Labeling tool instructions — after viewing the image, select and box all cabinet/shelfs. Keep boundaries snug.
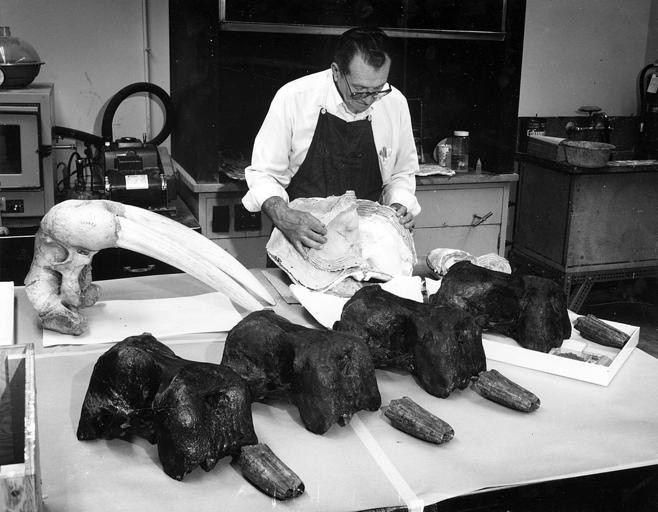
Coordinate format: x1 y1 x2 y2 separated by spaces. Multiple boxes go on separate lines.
171 158 516 278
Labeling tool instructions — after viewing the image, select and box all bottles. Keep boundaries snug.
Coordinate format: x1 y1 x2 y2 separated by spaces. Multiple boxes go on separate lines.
452 131 469 173
476 158 481 173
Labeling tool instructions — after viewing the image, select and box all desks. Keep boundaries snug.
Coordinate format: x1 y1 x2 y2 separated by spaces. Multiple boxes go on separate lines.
30 255 658 512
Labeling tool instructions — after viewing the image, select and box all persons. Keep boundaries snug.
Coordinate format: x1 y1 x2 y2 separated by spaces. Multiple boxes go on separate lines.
242 25 422 269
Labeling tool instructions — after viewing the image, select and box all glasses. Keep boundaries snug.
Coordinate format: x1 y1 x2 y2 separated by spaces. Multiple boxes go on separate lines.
342 69 392 101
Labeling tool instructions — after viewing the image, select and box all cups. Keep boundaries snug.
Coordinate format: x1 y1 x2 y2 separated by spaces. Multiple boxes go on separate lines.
436 144 452 167
